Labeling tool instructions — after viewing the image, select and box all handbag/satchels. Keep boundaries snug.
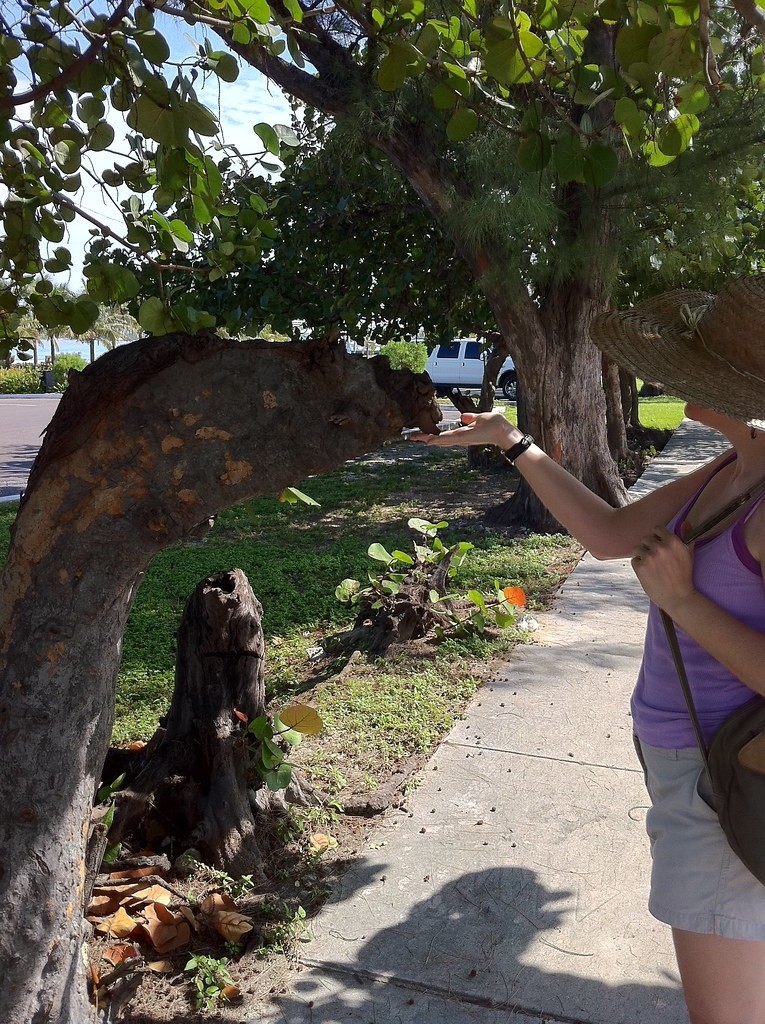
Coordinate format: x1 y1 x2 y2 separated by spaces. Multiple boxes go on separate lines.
706 694 765 887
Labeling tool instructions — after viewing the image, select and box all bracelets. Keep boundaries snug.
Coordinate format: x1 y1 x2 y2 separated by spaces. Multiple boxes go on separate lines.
501 435 534 466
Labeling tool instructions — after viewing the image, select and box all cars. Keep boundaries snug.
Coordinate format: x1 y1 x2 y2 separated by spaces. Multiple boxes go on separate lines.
424 339 517 400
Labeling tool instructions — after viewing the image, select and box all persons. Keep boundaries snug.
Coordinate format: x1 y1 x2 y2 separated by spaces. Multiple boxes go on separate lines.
405 274 764 1023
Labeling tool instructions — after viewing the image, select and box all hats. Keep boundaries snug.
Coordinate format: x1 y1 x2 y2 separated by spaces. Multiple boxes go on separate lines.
589 271 765 420
